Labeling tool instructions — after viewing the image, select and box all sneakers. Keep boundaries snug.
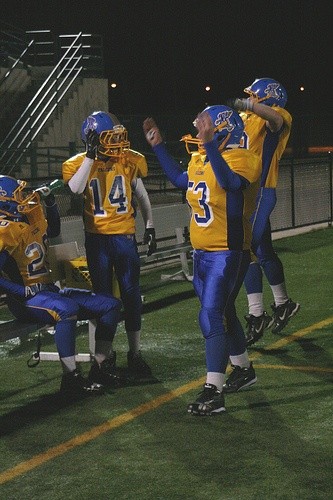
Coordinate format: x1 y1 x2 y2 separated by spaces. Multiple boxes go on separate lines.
126 349 153 376
60 370 105 395
186 383 226 417
270 297 301 334
87 359 128 388
245 311 274 348
220 360 257 392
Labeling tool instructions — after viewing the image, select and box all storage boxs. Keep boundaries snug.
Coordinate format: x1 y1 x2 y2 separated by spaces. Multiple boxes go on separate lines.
64 256 121 298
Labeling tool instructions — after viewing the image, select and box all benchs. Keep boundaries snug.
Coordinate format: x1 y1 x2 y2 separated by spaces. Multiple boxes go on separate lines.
0 243 147 361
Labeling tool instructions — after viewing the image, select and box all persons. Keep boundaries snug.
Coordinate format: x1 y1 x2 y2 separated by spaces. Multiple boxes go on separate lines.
62 111 157 378
142 106 262 415
226 78 301 344
0 175 129 398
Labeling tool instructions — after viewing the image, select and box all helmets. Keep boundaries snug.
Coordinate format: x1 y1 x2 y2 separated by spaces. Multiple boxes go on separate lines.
193 105 245 150
79 109 124 153
0 175 27 220
244 77 288 110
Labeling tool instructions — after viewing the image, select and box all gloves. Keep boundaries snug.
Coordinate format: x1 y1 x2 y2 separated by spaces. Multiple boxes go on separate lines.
206 97 254 113
37 182 56 206
23 282 62 298
141 227 157 258
85 127 100 160
